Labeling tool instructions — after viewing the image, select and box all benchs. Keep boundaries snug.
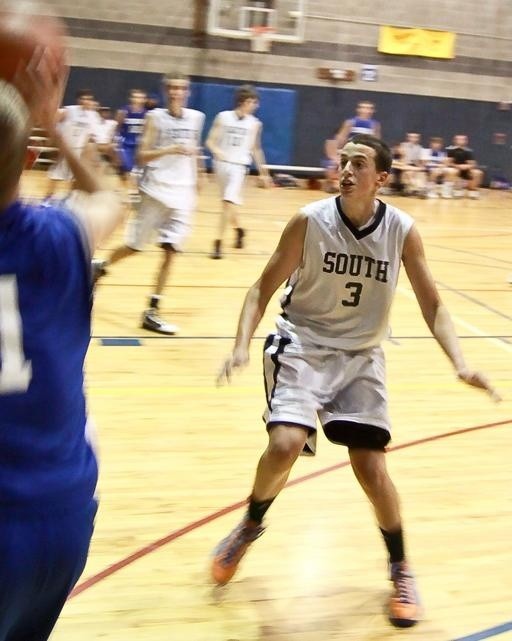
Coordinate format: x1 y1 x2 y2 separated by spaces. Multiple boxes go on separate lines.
26 124 214 174
259 163 329 191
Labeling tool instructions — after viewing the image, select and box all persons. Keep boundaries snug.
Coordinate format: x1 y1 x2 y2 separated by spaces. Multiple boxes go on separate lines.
0 47 127 641
40 71 206 335
328 100 482 199
206 86 273 259
209 133 503 626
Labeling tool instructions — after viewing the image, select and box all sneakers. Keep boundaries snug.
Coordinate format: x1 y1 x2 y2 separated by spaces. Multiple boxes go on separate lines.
385 562 424 629
210 250 222 260
209 517 266 588
141 308 180 335
91 259 106 293
233 230 246 249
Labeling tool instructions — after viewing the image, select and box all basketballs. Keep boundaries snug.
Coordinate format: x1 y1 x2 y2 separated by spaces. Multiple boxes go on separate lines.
0 4 68 107
